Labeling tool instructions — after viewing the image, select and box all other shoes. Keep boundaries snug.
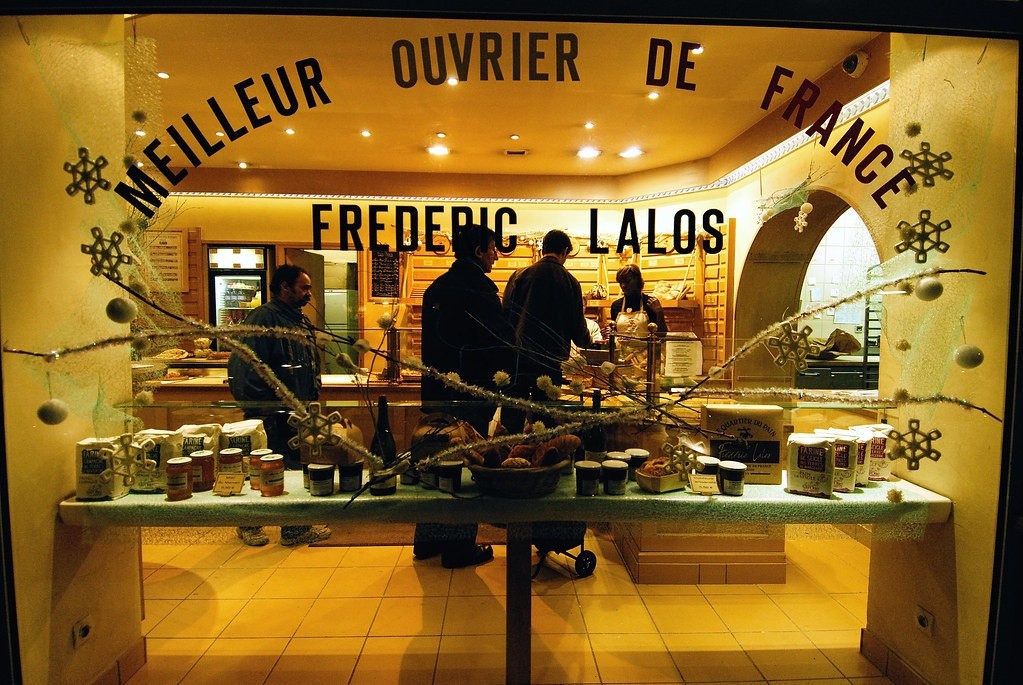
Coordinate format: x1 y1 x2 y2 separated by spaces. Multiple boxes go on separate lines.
234 526 269 546
280 524 331 546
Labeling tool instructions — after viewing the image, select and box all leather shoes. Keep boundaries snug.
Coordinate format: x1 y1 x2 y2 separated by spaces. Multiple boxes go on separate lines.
442 544 493 568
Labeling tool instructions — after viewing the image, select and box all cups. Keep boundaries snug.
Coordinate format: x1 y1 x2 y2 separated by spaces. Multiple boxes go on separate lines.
625 449 649 481
607 451 631 462
602 460 628 495
575 461 602 496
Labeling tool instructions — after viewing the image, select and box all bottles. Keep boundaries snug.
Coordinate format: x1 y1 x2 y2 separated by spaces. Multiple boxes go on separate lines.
719 461 747 496
400 467 418 485
583 390 608 484
259 454 284 497
438 461 463 492
225 283 262 308
249 449 273 490
420 465 438 490
303 464 334 496
219 448 243 474
338 464 363 492
368 396 396 496
166 456 193 499
696 455 721 475
595 340 608 350
190 450 214 492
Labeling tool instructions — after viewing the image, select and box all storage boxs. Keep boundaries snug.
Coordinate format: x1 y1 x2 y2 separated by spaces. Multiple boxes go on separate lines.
635 468 686 493
700 403 783 486
324 261 358 289
659 341 703 378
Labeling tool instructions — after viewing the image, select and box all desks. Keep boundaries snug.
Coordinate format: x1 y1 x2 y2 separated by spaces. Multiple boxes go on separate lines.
58 473 951 685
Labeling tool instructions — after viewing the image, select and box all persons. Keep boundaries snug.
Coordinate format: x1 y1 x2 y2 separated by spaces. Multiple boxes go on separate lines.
501 230 604 434
609 264 668 382
227 264 332 546
412 223 503 569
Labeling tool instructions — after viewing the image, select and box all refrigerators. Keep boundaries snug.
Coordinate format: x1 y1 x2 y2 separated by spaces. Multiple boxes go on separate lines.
324 289 359 374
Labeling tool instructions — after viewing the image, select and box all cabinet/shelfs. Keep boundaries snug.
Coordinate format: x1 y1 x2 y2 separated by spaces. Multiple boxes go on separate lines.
795 355 879 390
